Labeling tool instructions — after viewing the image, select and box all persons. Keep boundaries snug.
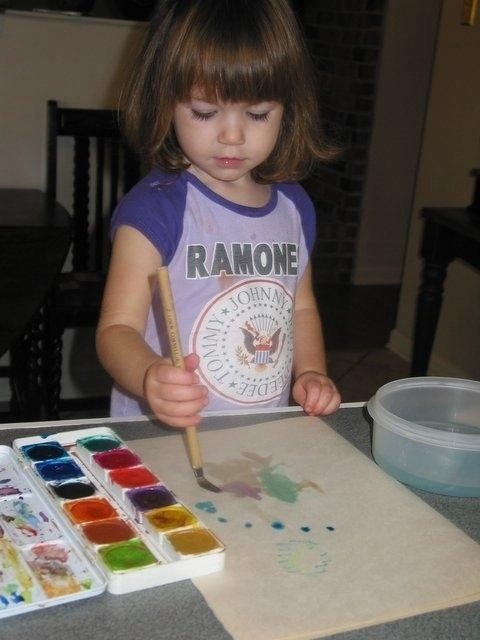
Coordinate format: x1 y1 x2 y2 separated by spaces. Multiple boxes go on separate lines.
94 0 345 429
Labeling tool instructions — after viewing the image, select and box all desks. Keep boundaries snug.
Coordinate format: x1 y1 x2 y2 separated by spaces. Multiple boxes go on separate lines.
0 188 72 420
0 393 480 640
410 169 480 379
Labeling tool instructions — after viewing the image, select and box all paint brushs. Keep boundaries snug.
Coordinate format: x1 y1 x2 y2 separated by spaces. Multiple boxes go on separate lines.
156 265 225 493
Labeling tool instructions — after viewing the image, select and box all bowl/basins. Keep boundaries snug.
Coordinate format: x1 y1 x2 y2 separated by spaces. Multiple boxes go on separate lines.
367 377 480 497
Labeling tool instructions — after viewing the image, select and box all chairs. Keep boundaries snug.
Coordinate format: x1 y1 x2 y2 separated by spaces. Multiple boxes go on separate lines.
29 100 170 420
